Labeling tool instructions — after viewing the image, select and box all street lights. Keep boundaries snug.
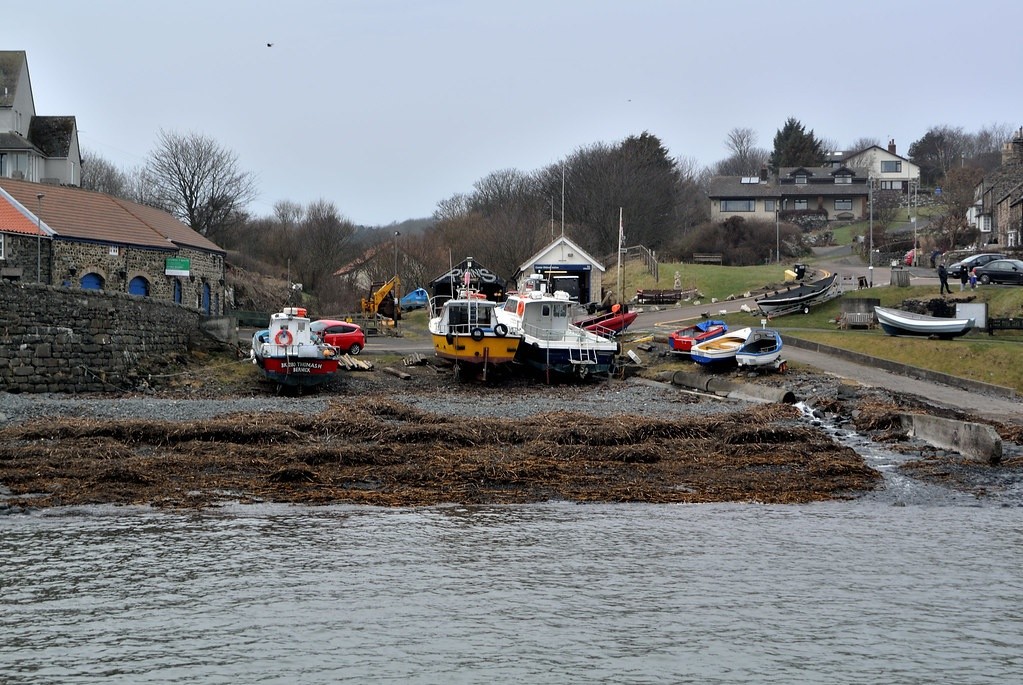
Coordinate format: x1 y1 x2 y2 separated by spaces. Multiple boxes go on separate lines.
909 181 917 266
868 179 875 287
37 193 45 282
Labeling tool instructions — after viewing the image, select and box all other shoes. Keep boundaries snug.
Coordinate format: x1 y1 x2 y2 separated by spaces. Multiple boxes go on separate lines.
941 293 946 295
948 292 954 294
971 289 973 291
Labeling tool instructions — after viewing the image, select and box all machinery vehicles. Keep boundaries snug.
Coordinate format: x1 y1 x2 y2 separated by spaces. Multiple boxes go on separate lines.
359 275 402 334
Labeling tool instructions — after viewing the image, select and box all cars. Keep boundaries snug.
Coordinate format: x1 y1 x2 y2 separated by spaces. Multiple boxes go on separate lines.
972 259 1023 284
309 320 364 355
946 253 1009 279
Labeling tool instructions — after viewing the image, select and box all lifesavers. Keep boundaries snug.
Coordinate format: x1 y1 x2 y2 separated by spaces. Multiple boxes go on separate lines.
275 331 293 348
471 328 484 341
494 323 508 338
446 333 454 345
517 301 524 315
471 294 486 299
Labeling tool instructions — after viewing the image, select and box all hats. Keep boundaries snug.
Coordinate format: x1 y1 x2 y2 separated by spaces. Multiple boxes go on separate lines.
971 268 976 272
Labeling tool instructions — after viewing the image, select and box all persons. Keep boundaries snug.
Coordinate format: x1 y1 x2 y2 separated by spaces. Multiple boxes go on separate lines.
959 263 977 292
988 238 998 244
938 263 953 295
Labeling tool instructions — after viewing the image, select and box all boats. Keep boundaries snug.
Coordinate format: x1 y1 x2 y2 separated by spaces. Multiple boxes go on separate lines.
491 292 620 379
690 327 753 368
735 327 782 367
251 307 340 390
429 271 521 378
758 272 837 319
573 311 637 336
668 319 728 354
873 306 975 338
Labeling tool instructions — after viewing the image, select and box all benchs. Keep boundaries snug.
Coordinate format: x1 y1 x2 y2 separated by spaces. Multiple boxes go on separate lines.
840 312 874 331
706 346 721 350
638 290 682 304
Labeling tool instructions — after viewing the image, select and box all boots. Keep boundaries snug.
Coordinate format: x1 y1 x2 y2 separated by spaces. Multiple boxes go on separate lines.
960 284 966 291
